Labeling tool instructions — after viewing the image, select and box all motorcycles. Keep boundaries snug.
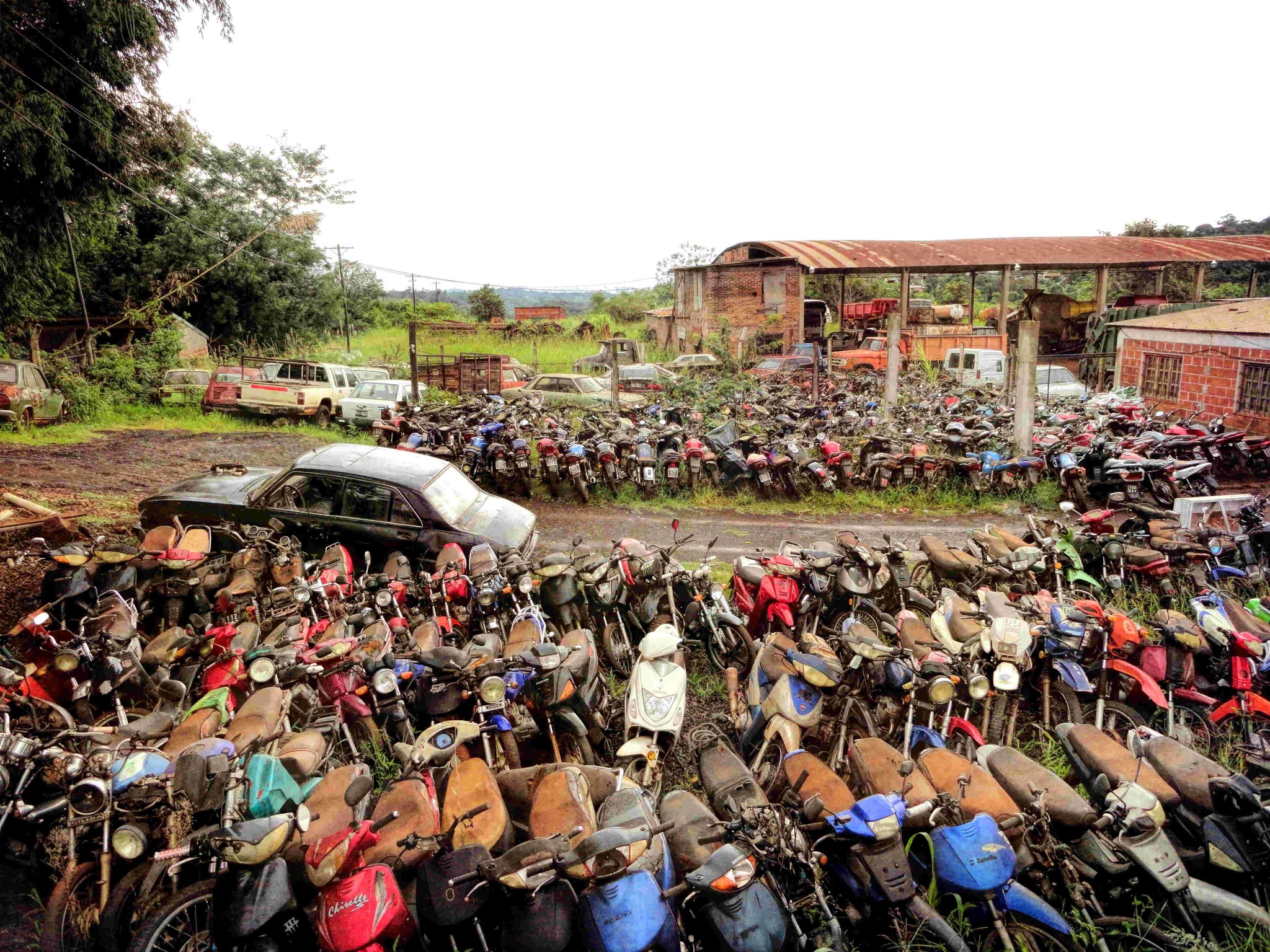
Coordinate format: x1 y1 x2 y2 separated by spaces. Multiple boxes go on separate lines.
0 345 1269 952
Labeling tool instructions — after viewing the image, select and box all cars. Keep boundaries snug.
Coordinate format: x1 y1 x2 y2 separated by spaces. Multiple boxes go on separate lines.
741 353 814 379
673 353 717 362
498 372 650 414
1035 363 1091 400
200 363 268 419
137 442 540 576
0 357 69 432
349 366 389 387
593 362 685 393
782 343 823 373
157 367 214 411
333 377 429 432
259 363 281 380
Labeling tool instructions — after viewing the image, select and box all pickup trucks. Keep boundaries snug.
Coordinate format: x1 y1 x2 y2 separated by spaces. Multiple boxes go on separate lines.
234 360 361 431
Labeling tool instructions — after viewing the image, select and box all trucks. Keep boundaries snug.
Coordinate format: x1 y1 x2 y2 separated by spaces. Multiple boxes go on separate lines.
941 347 1007 391
417 351 543 401
570 336 756 382
825 328 1009 376
804 299 828 343
838 297 899 333
